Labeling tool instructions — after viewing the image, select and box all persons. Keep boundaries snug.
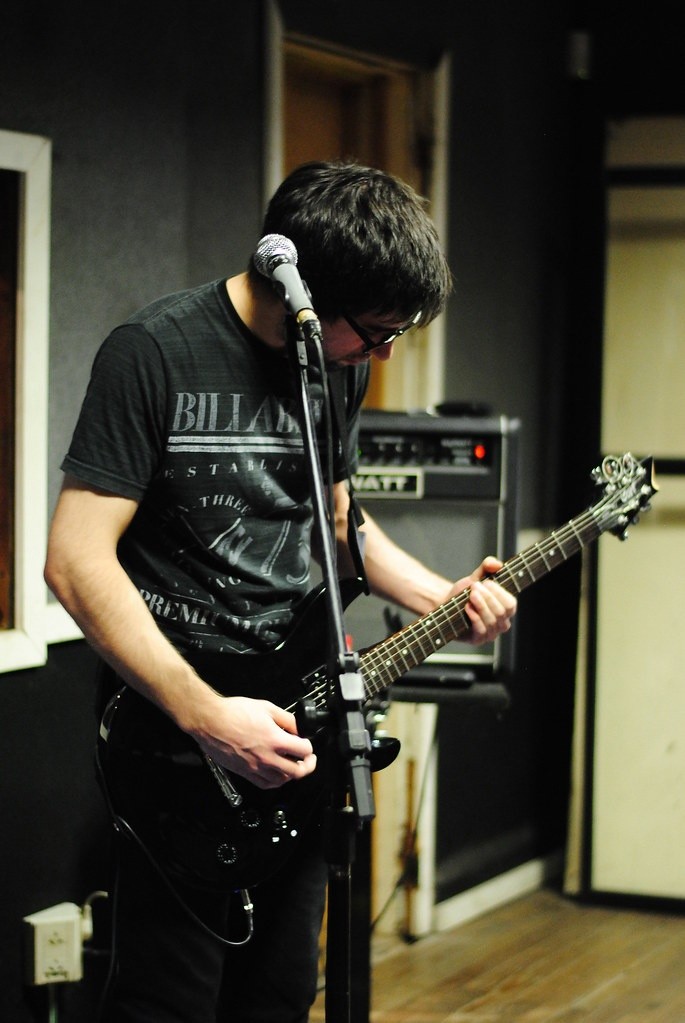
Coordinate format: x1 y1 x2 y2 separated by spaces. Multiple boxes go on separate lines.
42 161 517 1022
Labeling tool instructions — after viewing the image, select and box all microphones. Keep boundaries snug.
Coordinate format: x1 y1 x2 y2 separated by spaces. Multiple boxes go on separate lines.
253 234 321 340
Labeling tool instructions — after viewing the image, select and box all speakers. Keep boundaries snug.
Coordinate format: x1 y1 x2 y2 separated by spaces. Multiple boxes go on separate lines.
340 418 521 686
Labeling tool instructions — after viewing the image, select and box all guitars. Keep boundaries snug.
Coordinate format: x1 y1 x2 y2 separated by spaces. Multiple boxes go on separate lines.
96 448 663 950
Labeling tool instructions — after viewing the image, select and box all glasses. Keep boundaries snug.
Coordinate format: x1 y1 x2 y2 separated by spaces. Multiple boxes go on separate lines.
337 309 423 354
